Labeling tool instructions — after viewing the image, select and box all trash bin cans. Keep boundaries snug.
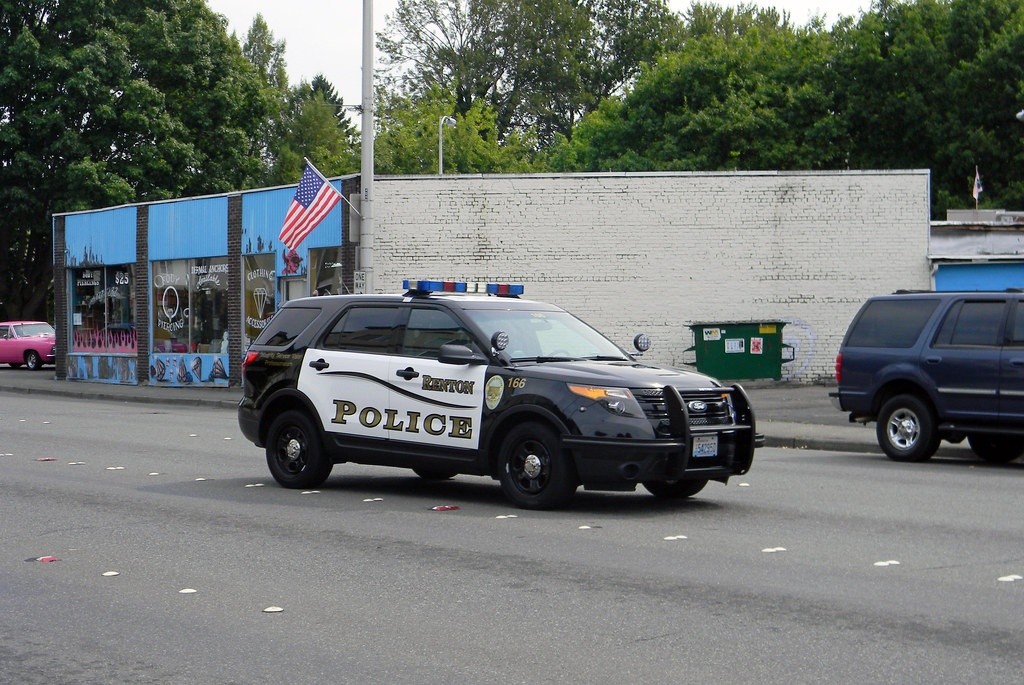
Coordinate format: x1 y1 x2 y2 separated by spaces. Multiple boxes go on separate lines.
682 321 794 381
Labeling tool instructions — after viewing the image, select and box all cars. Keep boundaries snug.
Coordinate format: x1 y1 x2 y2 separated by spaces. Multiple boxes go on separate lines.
0 320 56 372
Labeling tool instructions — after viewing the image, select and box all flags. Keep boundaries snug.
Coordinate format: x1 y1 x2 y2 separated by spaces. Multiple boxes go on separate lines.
278 165 343 252
972 170 983 198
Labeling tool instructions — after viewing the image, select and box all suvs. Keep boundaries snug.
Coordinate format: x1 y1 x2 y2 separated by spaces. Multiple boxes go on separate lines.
830 286 1024 461
236 280 764 511
